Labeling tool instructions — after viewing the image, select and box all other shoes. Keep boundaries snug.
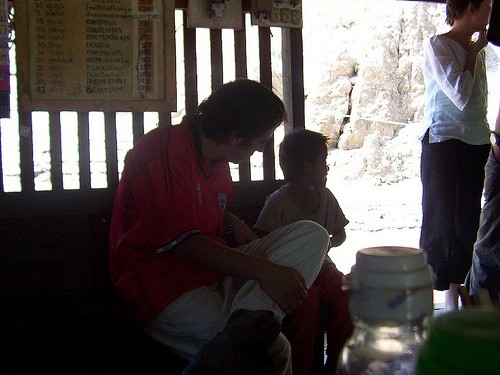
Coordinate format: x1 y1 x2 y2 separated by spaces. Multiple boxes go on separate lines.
182 336 235 375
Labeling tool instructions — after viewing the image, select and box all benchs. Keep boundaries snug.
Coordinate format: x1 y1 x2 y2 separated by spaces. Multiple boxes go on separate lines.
0 214 328 375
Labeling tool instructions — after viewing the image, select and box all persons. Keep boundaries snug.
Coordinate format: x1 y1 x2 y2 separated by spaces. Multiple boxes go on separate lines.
420 0 494 313
109 78 330 375
465 108 500 307
254 129 355 375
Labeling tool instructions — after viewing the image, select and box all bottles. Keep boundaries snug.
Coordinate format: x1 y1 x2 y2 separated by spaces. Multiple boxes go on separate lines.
336 246 434 375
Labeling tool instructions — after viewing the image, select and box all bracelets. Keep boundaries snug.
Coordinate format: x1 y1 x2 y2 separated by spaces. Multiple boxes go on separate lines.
227 217 238 234
